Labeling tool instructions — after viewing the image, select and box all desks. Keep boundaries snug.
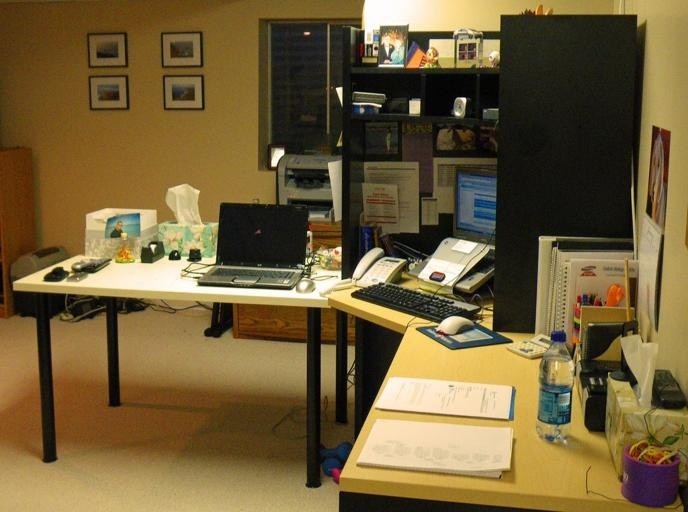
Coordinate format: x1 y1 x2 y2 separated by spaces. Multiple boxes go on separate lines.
11 254 349 489
329 272 495 439
338 326 687 512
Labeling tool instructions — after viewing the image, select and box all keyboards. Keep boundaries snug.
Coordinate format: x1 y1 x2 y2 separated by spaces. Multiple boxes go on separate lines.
351 281 481 323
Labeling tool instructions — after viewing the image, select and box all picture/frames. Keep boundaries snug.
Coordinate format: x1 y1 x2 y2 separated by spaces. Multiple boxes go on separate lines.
161 32 203 68
431 122 483 158
87 32 127 67
89 75 130 110
163 75 205 110
454 30 483 68
364 121 403 162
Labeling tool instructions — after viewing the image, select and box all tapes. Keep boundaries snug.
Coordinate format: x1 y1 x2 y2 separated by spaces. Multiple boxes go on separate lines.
150 244 157 256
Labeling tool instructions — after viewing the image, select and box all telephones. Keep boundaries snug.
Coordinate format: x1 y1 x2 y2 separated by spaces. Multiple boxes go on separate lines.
351 247 408 287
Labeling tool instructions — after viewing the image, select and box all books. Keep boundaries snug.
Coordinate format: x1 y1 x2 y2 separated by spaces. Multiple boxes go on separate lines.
535 236 639 345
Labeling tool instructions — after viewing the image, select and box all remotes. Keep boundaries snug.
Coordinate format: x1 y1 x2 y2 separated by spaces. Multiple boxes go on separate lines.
71 258 95 271
80 257 113 273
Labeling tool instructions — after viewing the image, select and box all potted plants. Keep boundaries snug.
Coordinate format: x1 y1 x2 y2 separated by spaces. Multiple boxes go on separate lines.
620 405 688 507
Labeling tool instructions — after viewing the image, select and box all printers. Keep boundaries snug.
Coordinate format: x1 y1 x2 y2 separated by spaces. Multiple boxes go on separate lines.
275 153 342 225
573 359 685 433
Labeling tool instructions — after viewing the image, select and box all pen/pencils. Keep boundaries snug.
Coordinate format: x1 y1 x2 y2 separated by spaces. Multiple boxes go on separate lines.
574 294 603 334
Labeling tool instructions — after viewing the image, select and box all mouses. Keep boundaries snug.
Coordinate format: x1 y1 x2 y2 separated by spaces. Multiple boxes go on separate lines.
435 315 476 335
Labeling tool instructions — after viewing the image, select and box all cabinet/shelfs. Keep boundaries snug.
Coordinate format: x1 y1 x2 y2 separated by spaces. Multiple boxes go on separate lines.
340 24 502 279
230 222 358 343
494 15 638 337
572 304 635 352
0 148 38 319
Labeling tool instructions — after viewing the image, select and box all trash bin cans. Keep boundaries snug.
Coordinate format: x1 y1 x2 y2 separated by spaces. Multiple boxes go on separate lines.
11 246 71 316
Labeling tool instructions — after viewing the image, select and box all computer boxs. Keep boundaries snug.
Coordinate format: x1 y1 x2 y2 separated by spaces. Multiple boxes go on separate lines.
353 316 405 442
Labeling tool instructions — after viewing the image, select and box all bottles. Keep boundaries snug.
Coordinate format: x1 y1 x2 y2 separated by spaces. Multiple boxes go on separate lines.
536 328 575 441
114 233 136 263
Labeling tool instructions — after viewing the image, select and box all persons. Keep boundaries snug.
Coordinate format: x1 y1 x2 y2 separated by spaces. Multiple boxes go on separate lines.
110 220 124 239
380 35 404 64
385 128 392 152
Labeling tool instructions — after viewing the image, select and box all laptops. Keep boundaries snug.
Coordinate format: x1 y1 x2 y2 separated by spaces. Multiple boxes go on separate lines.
407 165 498 294
198 201 310 290
